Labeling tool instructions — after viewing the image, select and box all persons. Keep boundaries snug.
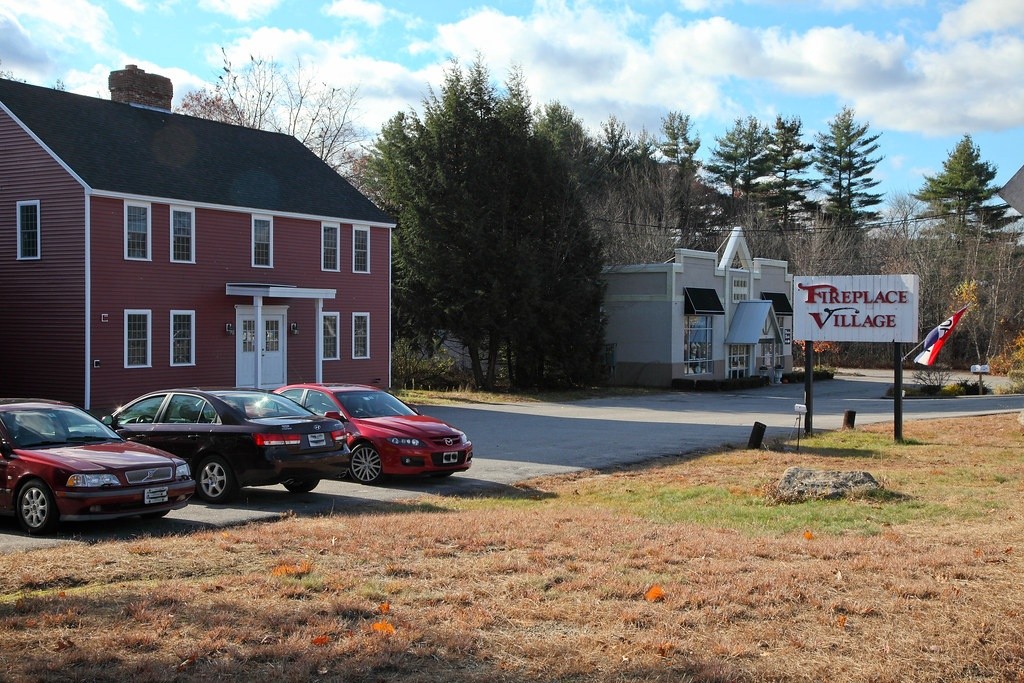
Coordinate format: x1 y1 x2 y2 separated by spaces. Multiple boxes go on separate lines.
173 398 198 425
305 391 335 416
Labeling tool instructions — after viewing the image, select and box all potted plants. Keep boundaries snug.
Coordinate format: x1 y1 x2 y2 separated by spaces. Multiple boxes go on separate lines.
759 366 768 376
774 364 784 383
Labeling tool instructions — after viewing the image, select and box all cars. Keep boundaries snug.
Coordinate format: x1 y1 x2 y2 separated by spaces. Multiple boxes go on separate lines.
0 397 196 538
239 382 474 486
95 386 354 506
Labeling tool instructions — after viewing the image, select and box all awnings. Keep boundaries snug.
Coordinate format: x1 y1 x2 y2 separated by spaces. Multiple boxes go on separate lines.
683 286 726 316
761 291 793 316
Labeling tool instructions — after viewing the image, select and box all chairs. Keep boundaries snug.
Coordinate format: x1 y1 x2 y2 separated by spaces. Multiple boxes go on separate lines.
346 397 360 408
311 395 322 404
177 400 198 419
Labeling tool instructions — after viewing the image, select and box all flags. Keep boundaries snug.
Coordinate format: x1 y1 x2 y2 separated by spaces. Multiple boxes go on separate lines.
913 305 969 368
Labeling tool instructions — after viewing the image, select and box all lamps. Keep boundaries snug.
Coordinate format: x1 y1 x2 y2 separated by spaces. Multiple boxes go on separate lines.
226 323 235 336
290 322 299 335
